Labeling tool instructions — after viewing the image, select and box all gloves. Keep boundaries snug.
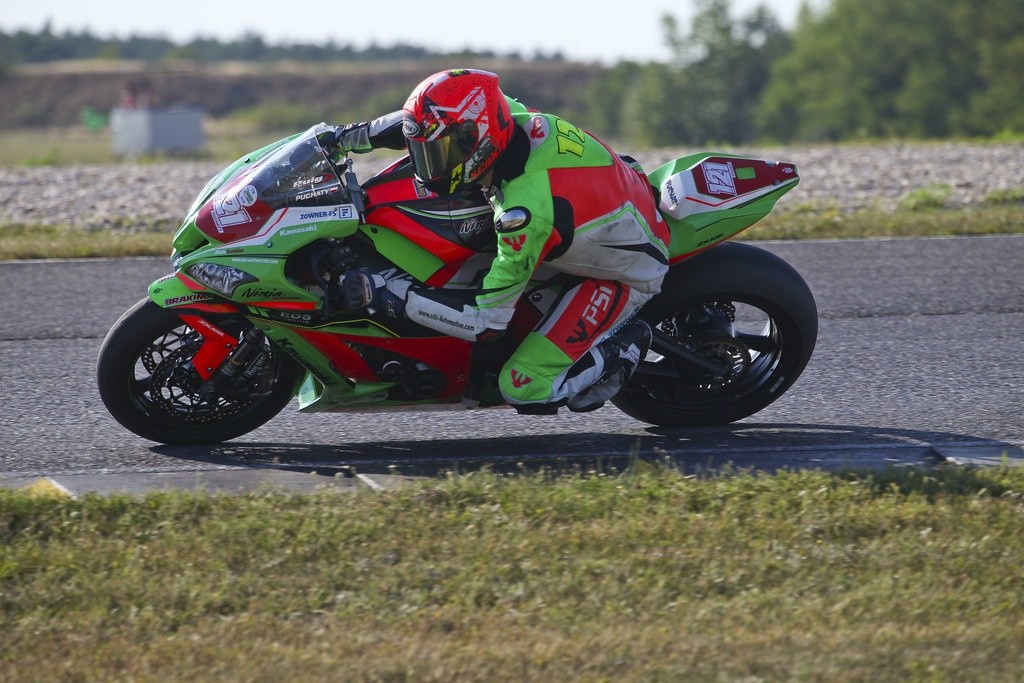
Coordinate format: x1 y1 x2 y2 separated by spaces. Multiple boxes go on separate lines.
329 271 411 320
289 120 372 177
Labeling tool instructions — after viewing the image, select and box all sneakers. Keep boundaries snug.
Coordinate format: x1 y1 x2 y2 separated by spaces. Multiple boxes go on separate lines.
567 320 652 410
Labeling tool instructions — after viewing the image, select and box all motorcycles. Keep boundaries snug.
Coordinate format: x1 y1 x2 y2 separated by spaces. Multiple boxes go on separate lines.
92 124 819 449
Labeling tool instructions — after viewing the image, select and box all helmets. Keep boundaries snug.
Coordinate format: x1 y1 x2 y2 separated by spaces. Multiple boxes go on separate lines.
402 68 513 196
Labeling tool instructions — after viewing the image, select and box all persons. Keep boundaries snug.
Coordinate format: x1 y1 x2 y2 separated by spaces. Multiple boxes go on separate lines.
309 68 670 409
